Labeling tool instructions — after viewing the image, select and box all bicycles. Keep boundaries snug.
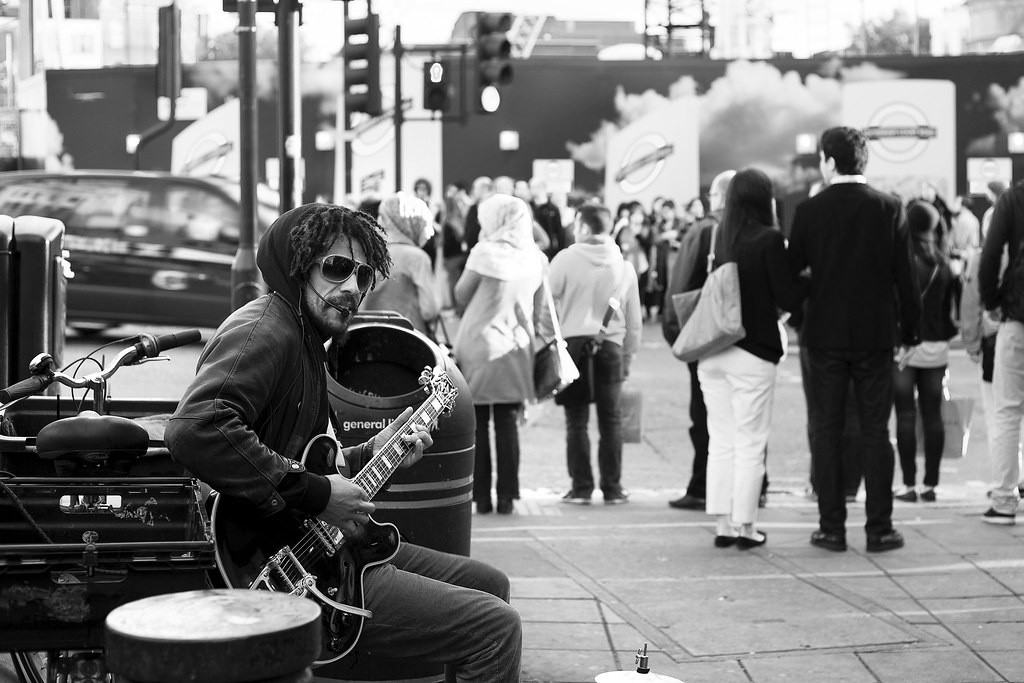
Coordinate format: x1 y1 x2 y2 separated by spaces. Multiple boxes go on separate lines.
0 329 215 683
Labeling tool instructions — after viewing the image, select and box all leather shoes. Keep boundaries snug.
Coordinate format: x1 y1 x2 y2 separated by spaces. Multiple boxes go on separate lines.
867 530 905 552
811 532 846 551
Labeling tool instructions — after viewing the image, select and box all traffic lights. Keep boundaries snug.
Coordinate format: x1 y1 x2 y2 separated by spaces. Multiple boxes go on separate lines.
473 12 515 115
346 15 381 116
423 62 448 111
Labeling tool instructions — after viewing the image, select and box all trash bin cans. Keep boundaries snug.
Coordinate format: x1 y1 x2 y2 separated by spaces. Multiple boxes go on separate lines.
324 310 475 556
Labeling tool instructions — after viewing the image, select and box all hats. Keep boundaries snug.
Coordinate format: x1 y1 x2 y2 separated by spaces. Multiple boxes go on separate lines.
907 201 939 241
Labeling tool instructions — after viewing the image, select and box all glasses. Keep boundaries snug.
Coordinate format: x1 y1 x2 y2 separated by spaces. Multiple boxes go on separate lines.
316 254 374 293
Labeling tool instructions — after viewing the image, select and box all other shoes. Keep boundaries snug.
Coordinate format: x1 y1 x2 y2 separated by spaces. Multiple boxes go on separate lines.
921 490 935 501
561 491 591 503
805 488 818 500
895 490 917 502
670 495 706 510
716 536 738 547
982 507 1015 524
605 490 630 503
737 531 767 549
497 499 512 513
846 495 856 502
477 500 492 514
988 489 1024 498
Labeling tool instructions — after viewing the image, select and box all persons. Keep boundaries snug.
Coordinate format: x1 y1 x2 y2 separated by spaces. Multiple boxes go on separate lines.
547 204 644 506
978 171 1024 525
164 202 523 682
803 178 863 503
662 168 770 511
414 180 705 322
788 125 923 552
453 193 548 516
892 198 959 503
696 169 795 551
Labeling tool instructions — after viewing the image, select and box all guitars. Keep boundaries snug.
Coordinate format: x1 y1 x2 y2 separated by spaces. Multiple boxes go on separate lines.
188 363 460 674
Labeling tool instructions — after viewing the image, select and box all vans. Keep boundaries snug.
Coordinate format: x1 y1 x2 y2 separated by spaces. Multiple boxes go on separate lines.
0 170 281 334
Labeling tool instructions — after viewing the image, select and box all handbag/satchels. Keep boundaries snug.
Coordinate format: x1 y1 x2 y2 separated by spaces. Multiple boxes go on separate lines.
620 391 643 443
535 336 580 403
672 223 746 362
553 341 597 405
